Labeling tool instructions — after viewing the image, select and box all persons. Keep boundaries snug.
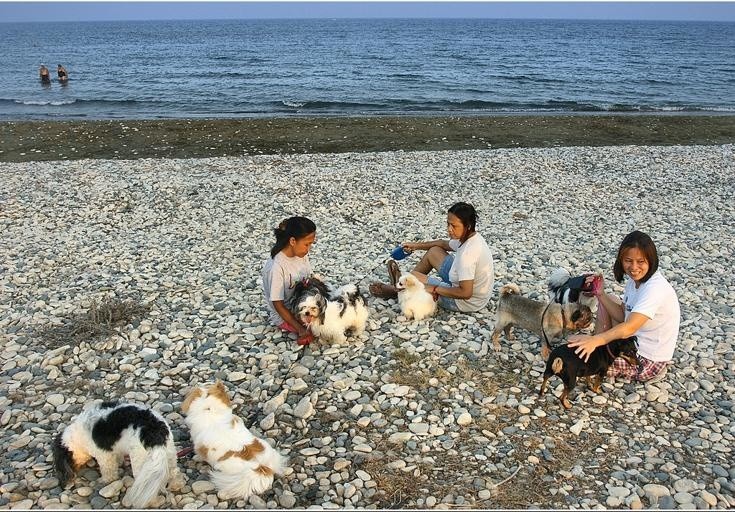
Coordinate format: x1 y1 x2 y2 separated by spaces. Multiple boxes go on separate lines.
566 230 681 382
368 201 495 313
262 216 317 347
57 64 68 80
39 64 50 81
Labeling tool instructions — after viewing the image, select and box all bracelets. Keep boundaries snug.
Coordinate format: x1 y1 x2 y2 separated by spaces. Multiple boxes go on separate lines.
432 285 440 302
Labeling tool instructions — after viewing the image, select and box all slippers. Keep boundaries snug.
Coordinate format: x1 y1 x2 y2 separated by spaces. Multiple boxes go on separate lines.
387 260 402 286
369 283 397 299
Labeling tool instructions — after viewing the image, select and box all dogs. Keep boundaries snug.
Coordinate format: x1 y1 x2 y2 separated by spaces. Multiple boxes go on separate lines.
539 329 640 409
392 272 437 320
546 262 599 314
179 381 285 501
489 281 593 363
49 399 187 509
289 276 370 346
